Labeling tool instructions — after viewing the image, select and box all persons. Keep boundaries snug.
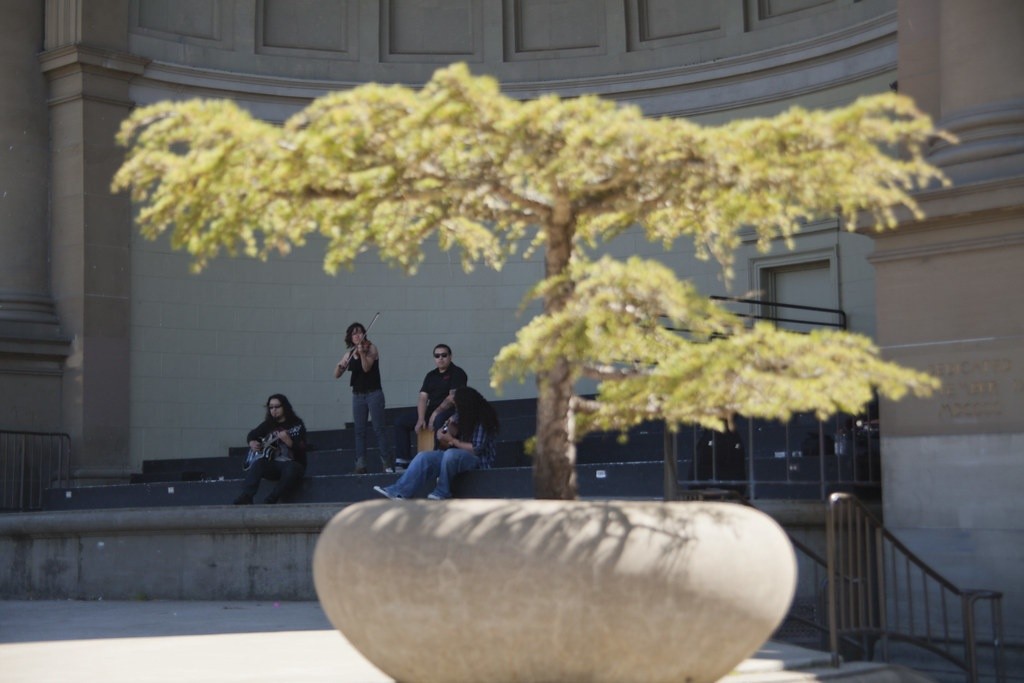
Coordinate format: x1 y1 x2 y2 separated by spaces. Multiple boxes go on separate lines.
386 344 468 472
335 323 393 473
373 386 498 500
233 394 307 505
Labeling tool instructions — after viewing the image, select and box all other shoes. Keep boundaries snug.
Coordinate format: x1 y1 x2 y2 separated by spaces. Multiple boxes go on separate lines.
232 492 254 504
373 485 404 501
428 493 445 500
277 493 301 503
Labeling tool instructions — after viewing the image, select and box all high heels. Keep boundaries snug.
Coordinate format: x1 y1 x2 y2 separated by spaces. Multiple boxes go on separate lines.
356 457 367 473
381 456 395 473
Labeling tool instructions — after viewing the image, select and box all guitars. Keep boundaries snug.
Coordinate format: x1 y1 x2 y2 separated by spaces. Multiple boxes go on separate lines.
242 424 304 472
439 421 460 450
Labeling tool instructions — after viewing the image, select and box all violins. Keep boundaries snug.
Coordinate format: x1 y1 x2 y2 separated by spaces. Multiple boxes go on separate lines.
355 340 372 356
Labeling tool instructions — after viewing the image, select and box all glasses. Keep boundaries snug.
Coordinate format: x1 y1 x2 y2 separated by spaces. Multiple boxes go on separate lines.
268 404 283 408
434 352 452 358
